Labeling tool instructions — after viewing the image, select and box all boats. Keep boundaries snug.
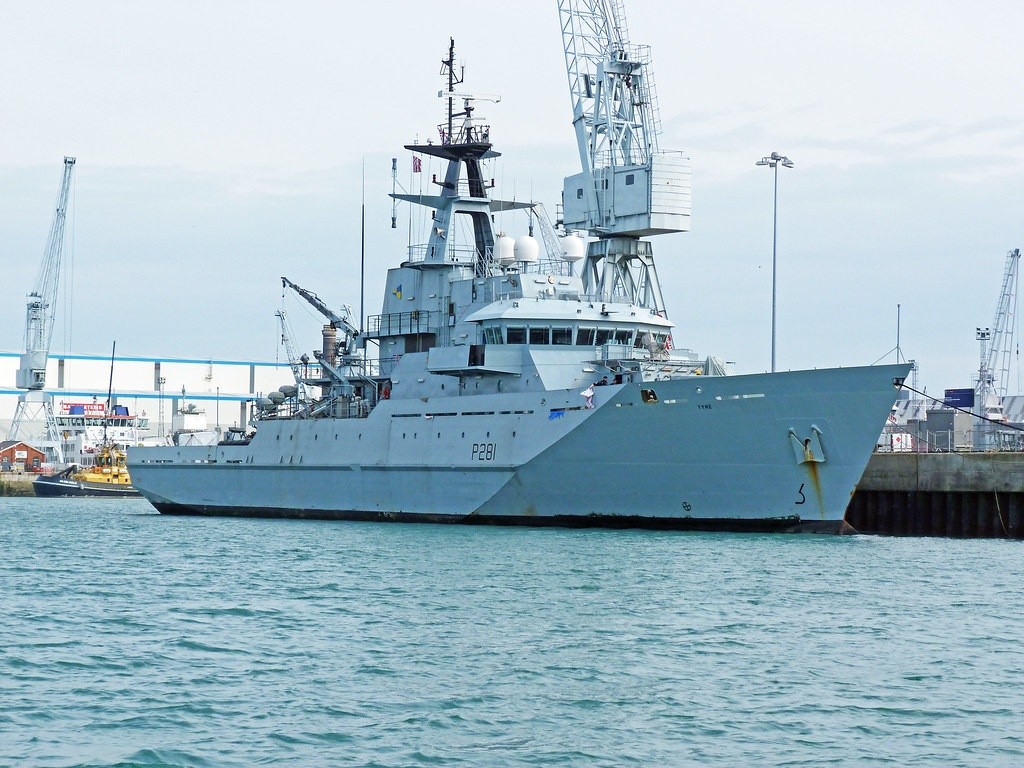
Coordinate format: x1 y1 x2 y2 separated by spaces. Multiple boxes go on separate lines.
853 402 1024 491
31 444 142 498
40 340 153 448
178 404 206 415
121 0 914 523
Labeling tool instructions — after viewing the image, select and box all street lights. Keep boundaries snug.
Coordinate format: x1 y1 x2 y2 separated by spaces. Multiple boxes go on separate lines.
180 384 188 434
755 151 797 371
215 386 221 432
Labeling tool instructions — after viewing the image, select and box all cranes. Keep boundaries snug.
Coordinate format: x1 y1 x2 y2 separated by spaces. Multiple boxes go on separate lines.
340 302 360 331
14 153 78 391
277 273 361 352
971 247 1023 423
271 304 304 384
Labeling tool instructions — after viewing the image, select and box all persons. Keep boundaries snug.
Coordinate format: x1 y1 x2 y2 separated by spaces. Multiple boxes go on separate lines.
593 376 608 386
383 387 390 399
610 379 618 385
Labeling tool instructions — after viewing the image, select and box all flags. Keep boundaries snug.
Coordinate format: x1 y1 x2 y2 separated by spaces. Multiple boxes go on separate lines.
392 284 401 299
413 156 421 173
666 334 672 350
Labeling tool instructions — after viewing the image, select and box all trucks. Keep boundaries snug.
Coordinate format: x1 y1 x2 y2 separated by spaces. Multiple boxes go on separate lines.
944 386 1007 422
